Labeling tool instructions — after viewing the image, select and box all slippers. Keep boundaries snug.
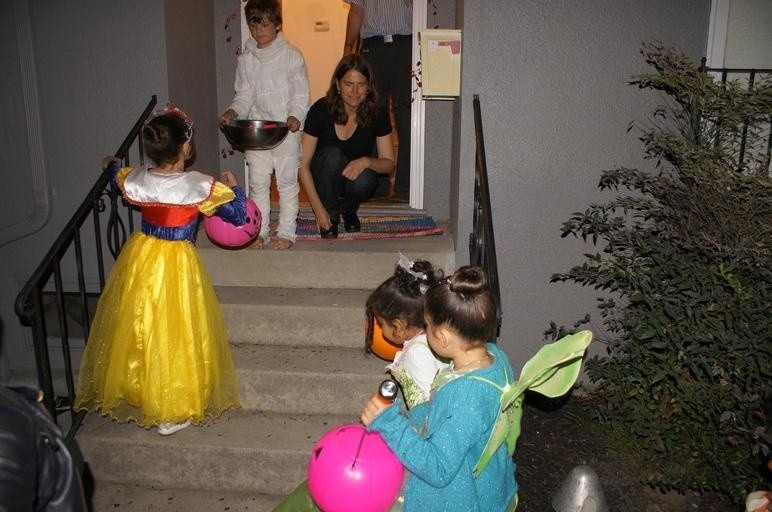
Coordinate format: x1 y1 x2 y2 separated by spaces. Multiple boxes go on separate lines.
275 238 294 250
250 237 266 249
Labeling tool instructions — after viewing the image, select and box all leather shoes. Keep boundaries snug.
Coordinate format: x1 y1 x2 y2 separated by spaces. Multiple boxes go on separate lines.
320 220 339 238
343 210 360 232
369 186 389 200
391 190 409 202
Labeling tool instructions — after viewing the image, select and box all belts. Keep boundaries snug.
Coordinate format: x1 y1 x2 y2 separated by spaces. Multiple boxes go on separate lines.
362 35 411 44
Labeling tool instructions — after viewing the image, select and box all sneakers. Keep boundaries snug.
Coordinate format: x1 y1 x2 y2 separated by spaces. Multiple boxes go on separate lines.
157 417 192 435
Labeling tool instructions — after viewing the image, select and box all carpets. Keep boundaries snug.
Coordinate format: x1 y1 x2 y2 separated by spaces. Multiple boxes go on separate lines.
270 215 443 241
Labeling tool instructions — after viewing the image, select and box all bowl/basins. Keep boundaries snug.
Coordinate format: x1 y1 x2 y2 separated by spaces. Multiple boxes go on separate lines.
220 119 291 151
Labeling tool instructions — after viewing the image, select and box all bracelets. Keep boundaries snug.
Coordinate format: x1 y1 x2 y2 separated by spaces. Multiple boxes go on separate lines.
345 43 354 48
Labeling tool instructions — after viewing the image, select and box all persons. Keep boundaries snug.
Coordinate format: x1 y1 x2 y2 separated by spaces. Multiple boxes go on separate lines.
364 252 453 409
344 1 413 200
216 0 310 250
297 53 395 239
361 266 521 511
73 110 247 437
